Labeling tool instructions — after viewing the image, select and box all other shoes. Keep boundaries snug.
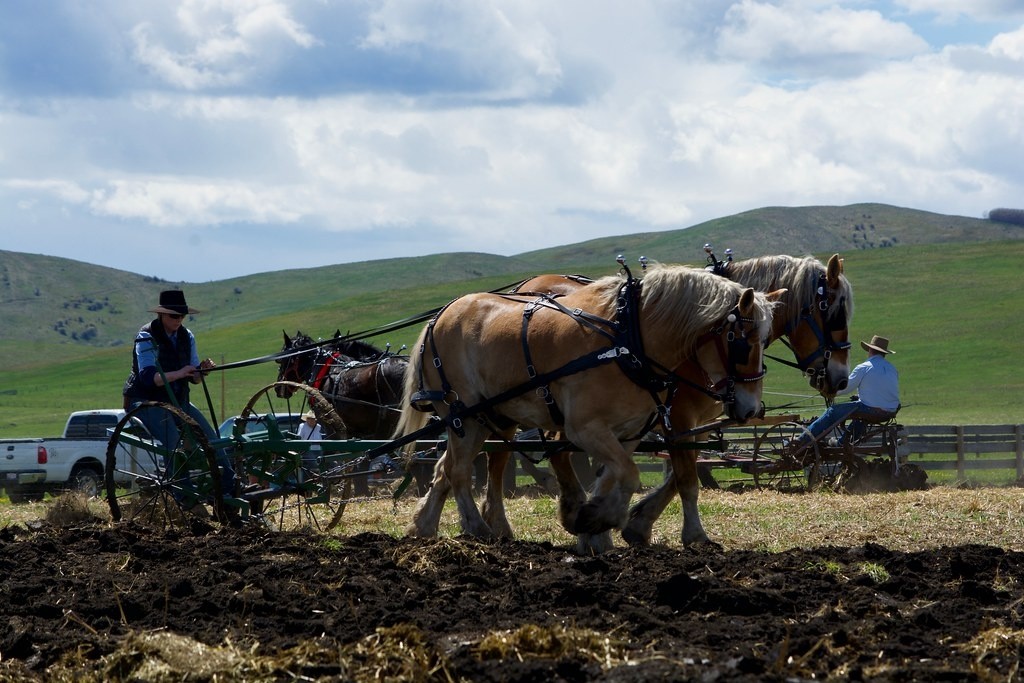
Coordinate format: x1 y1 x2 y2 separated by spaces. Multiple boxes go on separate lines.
828 436 843 447
788 436 807 448
231 481 262 498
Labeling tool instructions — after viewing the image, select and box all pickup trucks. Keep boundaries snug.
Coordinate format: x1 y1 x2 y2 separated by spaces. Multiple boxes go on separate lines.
0 407 174 505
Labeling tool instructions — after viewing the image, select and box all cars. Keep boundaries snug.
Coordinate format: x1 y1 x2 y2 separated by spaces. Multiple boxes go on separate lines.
215 413 308 459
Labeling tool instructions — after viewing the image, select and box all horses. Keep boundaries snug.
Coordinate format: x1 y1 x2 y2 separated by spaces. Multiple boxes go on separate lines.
273 329 562 498
389 252 854 557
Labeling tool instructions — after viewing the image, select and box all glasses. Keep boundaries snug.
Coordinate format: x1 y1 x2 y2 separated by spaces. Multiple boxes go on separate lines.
164 313 186 320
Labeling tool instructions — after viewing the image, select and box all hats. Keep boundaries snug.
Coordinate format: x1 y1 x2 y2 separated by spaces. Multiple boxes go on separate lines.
861 335 896 355
146 290 200 314
301 410 316 421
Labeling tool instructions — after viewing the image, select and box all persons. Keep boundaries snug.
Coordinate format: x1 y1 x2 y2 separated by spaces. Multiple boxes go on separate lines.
122 290 261 509
782 334 902 448
295 410 323 494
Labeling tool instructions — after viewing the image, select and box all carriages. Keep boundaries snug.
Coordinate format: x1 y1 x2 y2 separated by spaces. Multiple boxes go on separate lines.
274 325 900 498
100 241 852 552
